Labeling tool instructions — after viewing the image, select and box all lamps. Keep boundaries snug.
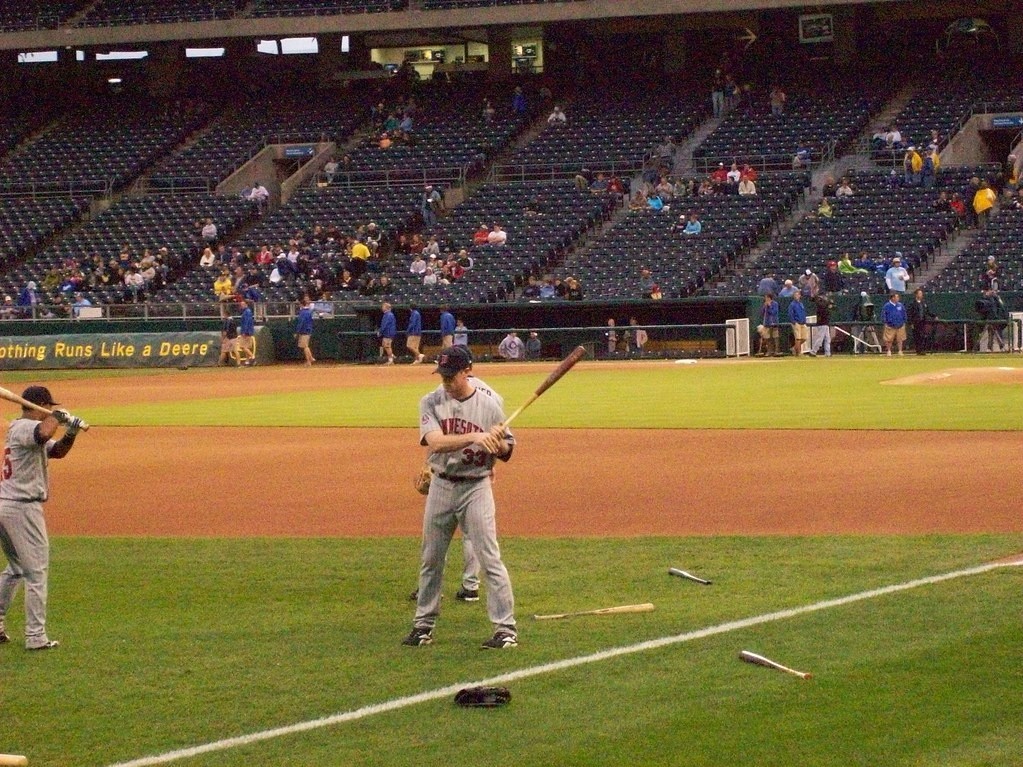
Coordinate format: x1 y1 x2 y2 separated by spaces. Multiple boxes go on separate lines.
513 45 523 55
422 50 432 60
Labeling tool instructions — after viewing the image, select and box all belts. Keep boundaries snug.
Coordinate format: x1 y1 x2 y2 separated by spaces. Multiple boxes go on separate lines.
431 469 487 482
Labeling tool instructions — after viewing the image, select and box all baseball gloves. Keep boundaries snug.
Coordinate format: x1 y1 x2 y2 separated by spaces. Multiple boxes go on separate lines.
453 685 512 708
414 465 432 495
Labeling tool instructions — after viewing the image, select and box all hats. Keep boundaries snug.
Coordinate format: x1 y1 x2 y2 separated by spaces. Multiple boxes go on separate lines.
161 247 167 252
458 250 466 256
987 256 995 260
830 261 836 269
680 215 684 220
430 254 436 259
564 277 573 283
432 346 469 376
456 344 473 364
20 386 59 406
805 269 812 276
5 296 11 301
893 258 900 263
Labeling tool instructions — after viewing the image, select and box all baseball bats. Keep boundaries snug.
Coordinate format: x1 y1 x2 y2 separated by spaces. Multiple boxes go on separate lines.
482 345 587 454
0 387 90 432
738 650 814 681
532 602 655 622
0 754 28 767
668 567 713 585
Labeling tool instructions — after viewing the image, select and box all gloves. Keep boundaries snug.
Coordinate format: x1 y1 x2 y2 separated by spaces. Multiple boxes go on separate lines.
52 409 70 426
65 416 84 435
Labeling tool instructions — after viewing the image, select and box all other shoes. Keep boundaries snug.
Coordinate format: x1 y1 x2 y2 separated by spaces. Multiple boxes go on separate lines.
35 641 57 650
0 632 10 642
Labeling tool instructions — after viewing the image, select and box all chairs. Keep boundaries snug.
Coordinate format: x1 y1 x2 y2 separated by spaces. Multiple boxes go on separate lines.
0 0 1023 321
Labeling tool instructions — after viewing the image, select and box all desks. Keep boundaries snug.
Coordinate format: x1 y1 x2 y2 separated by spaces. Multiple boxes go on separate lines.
512 56 538 75
410 61 440 80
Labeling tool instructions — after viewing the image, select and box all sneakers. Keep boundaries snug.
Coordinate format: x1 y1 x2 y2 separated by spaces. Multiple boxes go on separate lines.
401 627 432 648
456 588 479 601
482 631 517 649
410 587 420 599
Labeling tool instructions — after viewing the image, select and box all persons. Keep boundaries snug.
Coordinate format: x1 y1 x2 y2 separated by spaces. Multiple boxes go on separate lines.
440 306 455 349
640 269 663 299
622 315 648 352
604 319 620 353
526 329 540 361
0 386 85 650
757 125 1023 357
401 348 517 649
409 344 504 601
376 302 397 365
217 310 240 368
296 301 315 366
240 301 257 367
406 305 425 365
454 318 468 346
498 327 524 362
0 73 789 319
